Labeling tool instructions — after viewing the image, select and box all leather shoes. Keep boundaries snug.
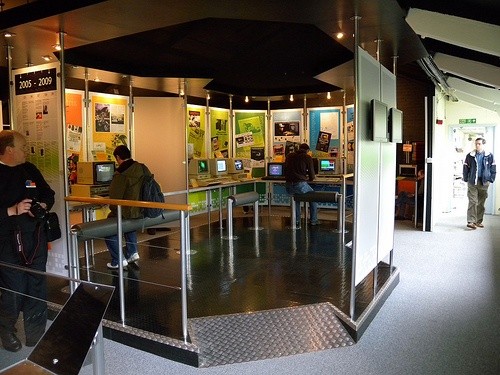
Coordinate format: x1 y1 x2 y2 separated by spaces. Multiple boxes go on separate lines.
25 332 45 346
0 328 22 351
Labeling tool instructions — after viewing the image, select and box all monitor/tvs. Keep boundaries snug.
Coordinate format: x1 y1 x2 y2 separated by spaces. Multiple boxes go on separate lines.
188 158 244 176
317 157 340 175
268 162 285 177
77 161 116 185
371 99 404 143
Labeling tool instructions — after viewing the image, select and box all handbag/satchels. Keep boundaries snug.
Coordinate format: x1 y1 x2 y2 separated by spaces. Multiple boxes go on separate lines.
140 163 165 220
40 198 62 241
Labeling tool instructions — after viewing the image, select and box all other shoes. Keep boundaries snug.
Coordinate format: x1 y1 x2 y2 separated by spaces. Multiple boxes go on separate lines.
475 222 484 228
156 228 171 231
127 253 140 262
311 219 322 225
147 229 155 235
107 259 128 268
467 223 476 229
296 222 301 229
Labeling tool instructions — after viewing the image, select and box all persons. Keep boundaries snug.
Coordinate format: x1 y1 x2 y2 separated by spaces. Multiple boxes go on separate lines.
395 170 425 220
276 124 288 136
284 144 320 229
462 137 496 230
105 145 151 269
0 131 56 352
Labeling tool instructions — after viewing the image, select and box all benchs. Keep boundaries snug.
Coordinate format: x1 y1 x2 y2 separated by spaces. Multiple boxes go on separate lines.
219 191 265 240
288 191 350 234
60 209 197 295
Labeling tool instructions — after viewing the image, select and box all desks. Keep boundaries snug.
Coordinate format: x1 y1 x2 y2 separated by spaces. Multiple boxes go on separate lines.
255 171 354 225
397 175 424 228
69 190 188 263
189 179 255 228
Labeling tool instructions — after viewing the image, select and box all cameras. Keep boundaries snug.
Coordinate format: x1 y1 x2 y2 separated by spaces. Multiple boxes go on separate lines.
29 198 46 219
